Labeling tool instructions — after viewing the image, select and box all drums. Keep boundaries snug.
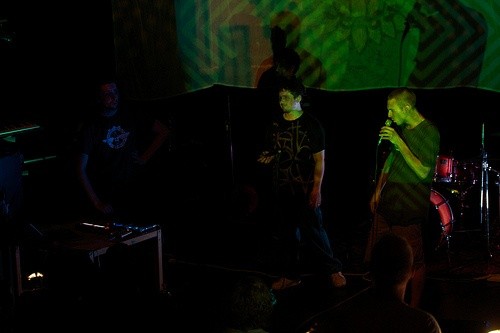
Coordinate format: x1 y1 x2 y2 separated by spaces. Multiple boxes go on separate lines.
430 186 463 250
434 153 460 192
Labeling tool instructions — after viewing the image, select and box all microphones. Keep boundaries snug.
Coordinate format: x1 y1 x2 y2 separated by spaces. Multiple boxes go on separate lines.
379 119 392 145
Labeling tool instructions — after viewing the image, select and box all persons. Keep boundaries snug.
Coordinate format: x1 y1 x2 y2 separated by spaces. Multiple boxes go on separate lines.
311 234 442 333
80 80 167 215
274 50 300 75
364 89 441 309
257 80 348 290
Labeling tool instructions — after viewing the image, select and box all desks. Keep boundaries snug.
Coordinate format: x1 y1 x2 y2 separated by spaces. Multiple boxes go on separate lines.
16 223 164 293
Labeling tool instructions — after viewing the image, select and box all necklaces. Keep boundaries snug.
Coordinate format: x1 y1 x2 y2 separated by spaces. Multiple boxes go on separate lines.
224 280 275 333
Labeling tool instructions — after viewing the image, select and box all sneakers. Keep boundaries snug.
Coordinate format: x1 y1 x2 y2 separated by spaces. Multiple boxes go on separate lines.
331 271 346 287
272 276 304 289
